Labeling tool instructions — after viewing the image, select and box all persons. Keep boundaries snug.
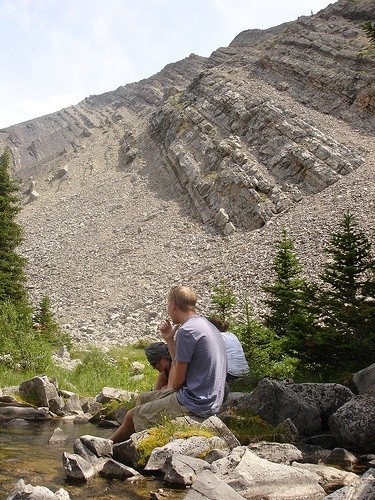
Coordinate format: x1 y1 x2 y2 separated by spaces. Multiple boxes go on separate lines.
110 286 228 444
207 317 250 383
145 342 172 390
